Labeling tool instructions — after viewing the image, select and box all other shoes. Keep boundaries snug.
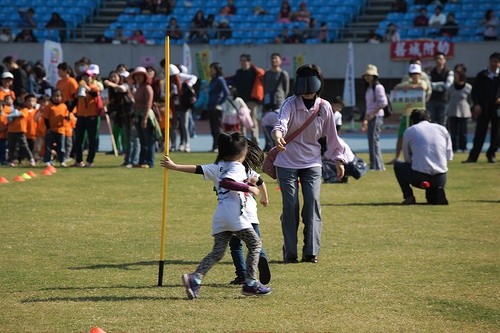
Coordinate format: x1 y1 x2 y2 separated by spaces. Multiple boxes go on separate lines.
106 143 191 168
400 196 416 204
9 159 94 167
436 186 448 205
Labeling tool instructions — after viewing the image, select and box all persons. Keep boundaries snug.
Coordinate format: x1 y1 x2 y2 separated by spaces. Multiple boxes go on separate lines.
369 0 499 44
160 131 271 300
361 64 388 171
97 0 236 44
273 0 329 44
394 109 453 205
385 53 500 165
0 8 66 44
271 65 345 264
0 55 200 168
206 54 291 153
321 96 355 183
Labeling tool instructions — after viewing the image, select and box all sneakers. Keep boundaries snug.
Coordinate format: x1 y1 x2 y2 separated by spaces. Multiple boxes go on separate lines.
301 254 317 263
182 273 201 299
229 278 242 284
258 257 271 285
242 280 271 296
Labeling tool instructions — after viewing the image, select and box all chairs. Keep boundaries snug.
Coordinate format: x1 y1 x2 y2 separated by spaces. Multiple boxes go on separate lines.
368 0 500 43
103 0 366 44
0 0 102 43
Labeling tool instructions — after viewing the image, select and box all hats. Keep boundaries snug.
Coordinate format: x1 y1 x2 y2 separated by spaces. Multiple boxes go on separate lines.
292 75 322 95
361 64 379 78
169 63 180 77
1 71 14 78
408 64 422 74
89 64 100 74
127 67 150 85
82 68 94 76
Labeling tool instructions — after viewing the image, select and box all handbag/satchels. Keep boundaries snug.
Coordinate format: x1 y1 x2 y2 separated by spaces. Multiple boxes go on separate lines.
183 83 198 106
237 108 253 128
347 154 369 180
262 146 277 179
